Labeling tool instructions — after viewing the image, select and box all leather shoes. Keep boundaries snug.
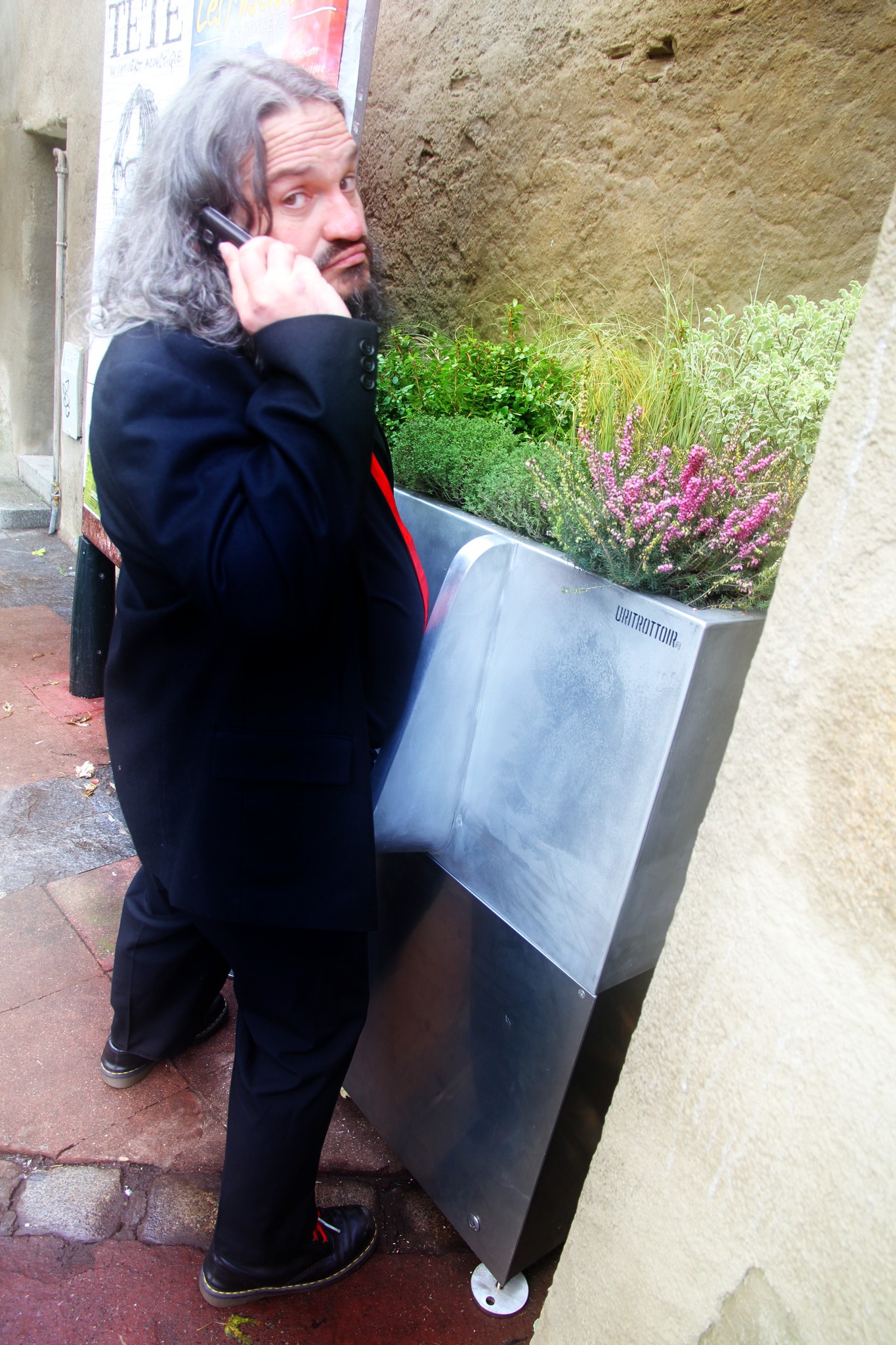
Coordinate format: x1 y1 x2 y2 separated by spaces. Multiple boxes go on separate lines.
100 992 228 1088
199 1204 378 1309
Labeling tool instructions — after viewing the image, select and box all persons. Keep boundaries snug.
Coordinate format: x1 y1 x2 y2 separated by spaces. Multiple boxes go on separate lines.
89 52 432 1308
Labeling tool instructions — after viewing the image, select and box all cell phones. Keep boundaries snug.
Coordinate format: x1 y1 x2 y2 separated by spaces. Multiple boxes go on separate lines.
198 206 258 274
